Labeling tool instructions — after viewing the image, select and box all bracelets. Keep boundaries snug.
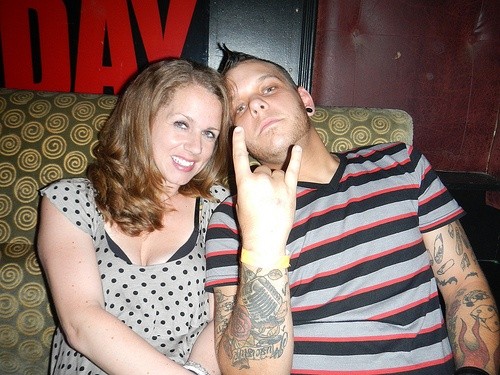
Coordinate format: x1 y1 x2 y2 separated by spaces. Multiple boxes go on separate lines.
240 245 292 269
182 358 211 375
453 366 491 375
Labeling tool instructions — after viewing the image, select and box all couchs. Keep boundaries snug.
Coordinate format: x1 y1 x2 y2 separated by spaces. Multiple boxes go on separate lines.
0 89 413 375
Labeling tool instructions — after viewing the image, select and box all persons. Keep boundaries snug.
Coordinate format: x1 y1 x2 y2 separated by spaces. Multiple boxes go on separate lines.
203 42 500 375
35 56 235 375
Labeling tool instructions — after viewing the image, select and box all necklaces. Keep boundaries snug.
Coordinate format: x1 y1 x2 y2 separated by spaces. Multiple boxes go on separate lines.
161 190 179 203
